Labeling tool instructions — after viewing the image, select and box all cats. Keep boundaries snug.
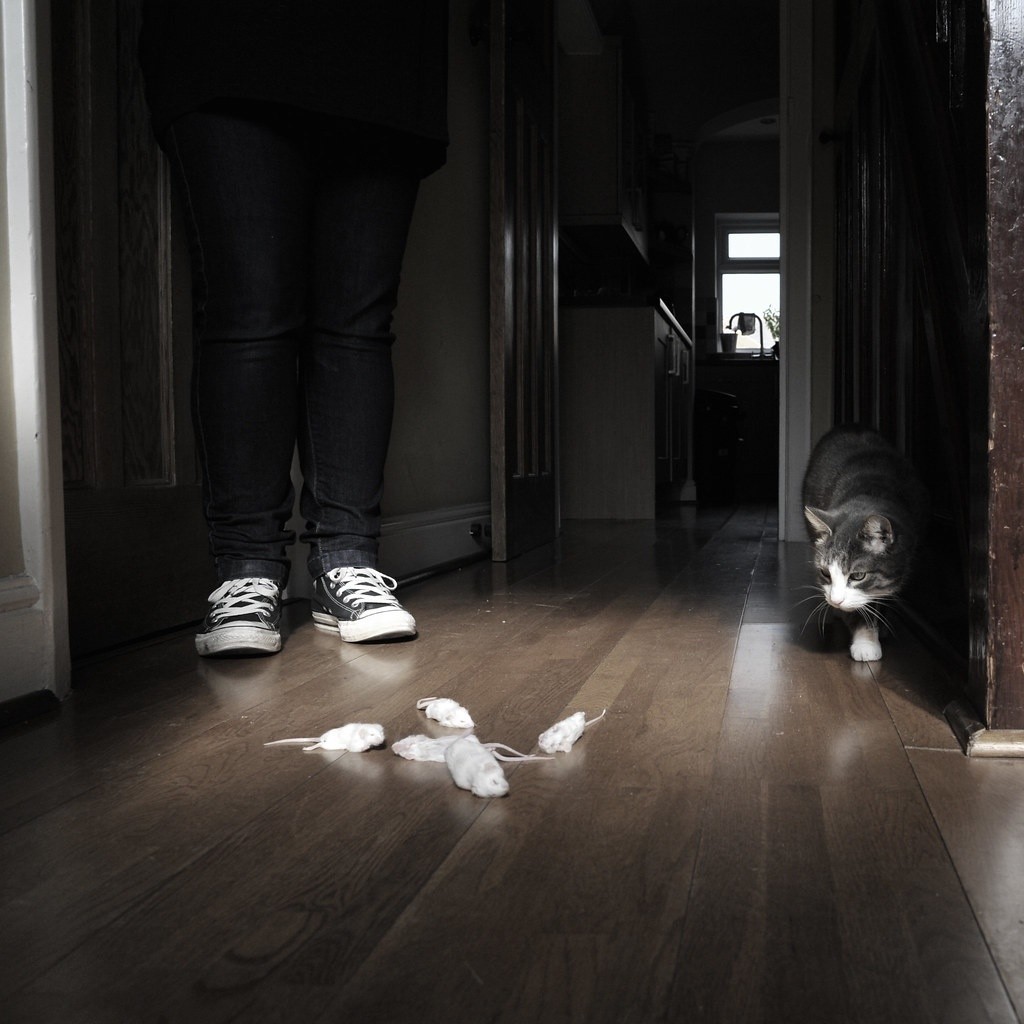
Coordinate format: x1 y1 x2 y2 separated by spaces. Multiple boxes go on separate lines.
788 419 934 662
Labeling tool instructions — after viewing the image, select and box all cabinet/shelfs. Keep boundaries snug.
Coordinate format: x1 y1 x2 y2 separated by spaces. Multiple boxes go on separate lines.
692 363 779 498
557 46 649 270
561 306 693 517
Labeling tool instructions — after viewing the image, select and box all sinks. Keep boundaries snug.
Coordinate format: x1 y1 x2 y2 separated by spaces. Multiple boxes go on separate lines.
724 356 775 360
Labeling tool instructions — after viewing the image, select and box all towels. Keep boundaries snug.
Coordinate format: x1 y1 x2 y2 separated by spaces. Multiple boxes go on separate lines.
738 312 754 335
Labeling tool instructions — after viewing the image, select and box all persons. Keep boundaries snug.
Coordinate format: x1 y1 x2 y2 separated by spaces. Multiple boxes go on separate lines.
141 0 450 658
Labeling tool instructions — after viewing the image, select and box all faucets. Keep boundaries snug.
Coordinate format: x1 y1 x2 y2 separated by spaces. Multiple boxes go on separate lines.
726 314 763 354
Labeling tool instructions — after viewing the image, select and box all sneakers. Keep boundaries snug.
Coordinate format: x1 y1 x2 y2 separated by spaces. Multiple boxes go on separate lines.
309 565 418 644
195 575 284 656
